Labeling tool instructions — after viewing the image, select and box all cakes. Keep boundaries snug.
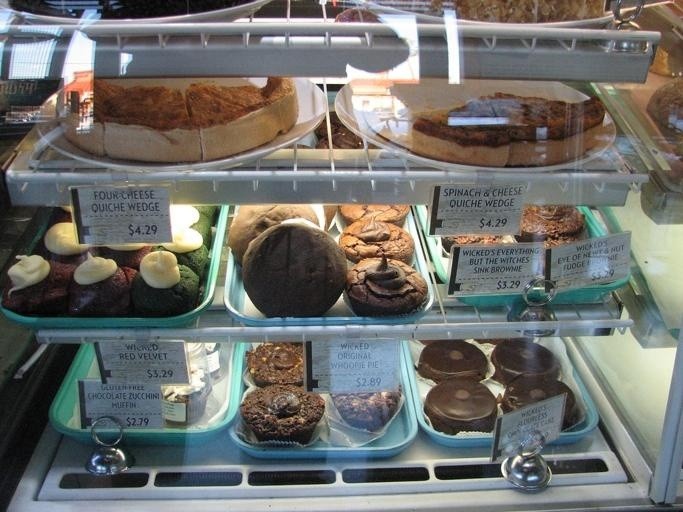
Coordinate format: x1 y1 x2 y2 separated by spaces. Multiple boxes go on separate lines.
2 204 220 317
161 343 221 428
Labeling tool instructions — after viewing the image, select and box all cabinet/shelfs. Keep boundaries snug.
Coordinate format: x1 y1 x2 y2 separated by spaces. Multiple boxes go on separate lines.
0 0 682 510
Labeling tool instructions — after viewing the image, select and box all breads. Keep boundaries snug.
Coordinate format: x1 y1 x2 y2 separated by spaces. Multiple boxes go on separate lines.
646 76 683 162
228 203 428 317
418 338 575 435
413 92 605 146
56 72 298 163
621 0 683 77
431 0 606 23
441 204 586 251
240 342 401 449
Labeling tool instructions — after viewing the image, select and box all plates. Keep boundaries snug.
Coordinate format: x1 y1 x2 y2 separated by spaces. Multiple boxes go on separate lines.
36 80 328 173
335 80 616 170
354 2 617 41
2 0 267 38
